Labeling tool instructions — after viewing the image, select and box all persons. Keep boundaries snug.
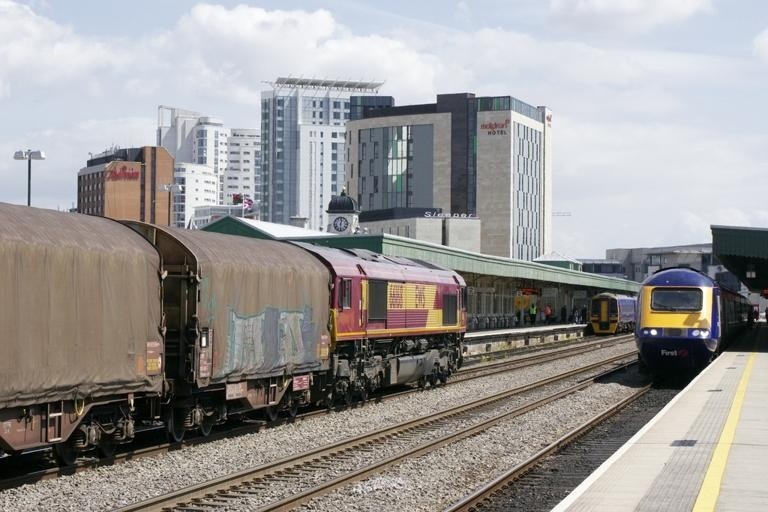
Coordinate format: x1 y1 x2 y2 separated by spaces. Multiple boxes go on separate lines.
542 302 553 325
528 303 539 326
580 307 587 324
558 305 567 323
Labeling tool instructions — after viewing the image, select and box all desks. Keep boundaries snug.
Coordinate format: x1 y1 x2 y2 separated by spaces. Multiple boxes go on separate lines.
13 148 47 206
